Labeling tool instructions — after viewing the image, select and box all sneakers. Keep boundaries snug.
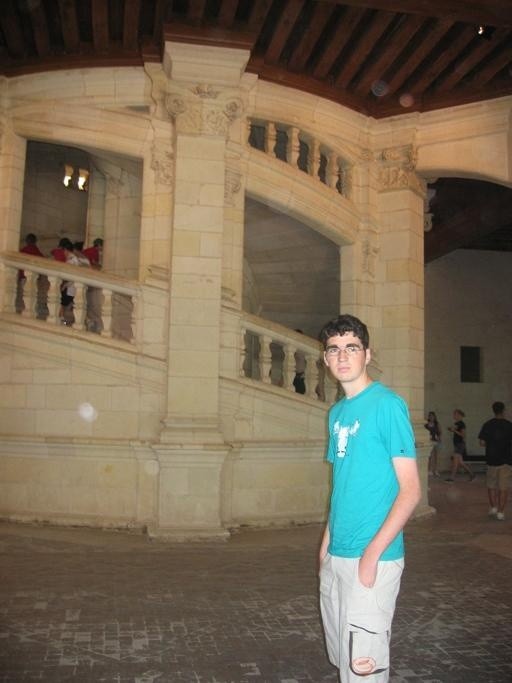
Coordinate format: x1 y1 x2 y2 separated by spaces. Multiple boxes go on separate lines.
488 507 505 521
468 475 477 482
445 478 454 484
428 470 440 479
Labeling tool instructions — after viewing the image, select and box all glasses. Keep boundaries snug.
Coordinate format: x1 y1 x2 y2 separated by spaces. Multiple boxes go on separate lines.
326 347 364 356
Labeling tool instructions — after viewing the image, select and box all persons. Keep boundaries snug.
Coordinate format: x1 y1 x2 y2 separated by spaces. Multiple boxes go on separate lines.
315 314 419 683
17 234 104 327
425 401 512 524
288 329 308 394
